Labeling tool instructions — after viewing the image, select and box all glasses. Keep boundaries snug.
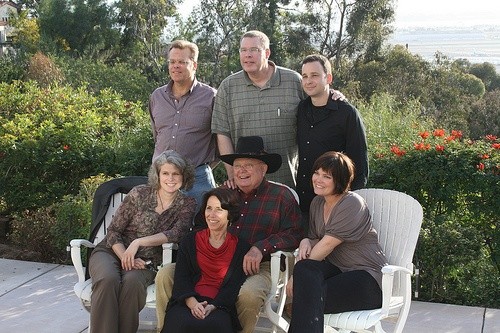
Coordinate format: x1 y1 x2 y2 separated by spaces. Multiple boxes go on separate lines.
239 47 259 55
231 162 263 172
167 59 190 66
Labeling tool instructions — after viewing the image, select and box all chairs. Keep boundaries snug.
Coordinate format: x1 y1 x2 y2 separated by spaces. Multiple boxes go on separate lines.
70 176 423 333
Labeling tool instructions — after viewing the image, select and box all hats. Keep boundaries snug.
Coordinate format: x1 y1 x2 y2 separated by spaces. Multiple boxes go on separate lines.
217 136 283 174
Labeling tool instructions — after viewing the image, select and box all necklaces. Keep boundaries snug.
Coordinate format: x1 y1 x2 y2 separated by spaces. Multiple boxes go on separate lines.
157 190 177 209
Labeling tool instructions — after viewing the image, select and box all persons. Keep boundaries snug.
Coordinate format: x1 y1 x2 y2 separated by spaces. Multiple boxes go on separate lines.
159 187 251 333
154 136 306 333
287 151 390 333
88 150 196 333
296 54 369 217
148 39 221 215
211 30 349 192
